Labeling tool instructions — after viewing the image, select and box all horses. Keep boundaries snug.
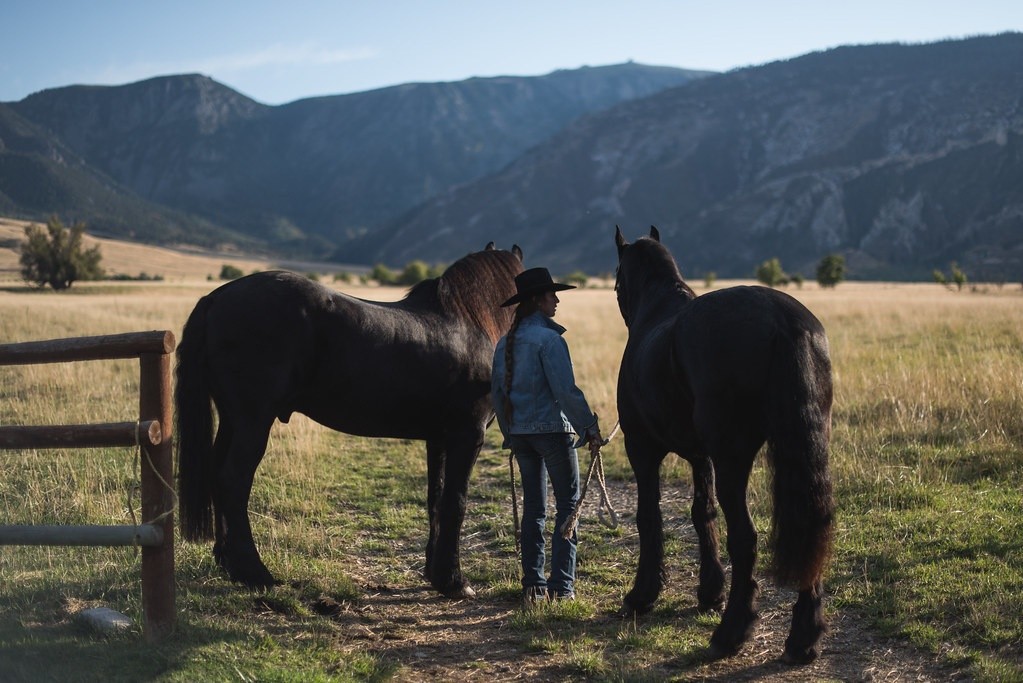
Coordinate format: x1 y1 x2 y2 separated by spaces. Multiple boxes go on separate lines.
174 240 527 601
610 223 838 666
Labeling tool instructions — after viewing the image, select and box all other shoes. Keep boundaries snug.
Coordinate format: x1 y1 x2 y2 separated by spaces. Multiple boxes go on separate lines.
533 590 576 603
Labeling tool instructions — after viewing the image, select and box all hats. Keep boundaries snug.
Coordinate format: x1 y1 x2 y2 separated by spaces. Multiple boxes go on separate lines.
501 267 577 308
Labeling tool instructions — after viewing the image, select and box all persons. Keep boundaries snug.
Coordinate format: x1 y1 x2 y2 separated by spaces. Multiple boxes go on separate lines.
488 268 604 612
